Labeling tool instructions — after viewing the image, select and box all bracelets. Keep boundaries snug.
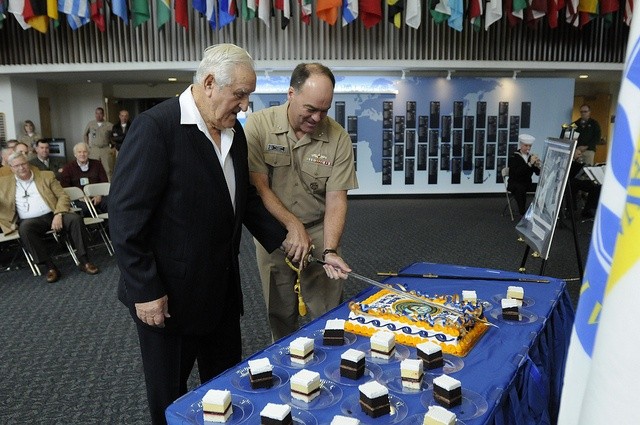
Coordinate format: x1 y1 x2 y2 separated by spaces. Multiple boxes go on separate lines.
322 249 338 261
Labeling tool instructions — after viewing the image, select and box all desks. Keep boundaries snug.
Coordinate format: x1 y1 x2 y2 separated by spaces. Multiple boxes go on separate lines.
165 261 576 425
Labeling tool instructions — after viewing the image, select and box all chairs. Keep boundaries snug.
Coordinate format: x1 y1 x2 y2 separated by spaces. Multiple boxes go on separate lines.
84 183 117 255
21 211 79 276
501 167 536 221
0 205 39 276
55 187 113 265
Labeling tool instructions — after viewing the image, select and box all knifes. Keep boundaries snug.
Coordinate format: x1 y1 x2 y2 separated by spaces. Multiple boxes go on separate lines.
278 243 501 330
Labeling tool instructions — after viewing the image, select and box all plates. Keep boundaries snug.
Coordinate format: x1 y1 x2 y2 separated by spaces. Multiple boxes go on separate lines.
382 369 436 396
411 353 465 375
419 389 488 420
492 293 536 309
230 365 290 393
323 359 383 387
401 412 463 425
273 346 327 369
307 329 358 350
279 409 319 425
355 341 410 365
489 310 540 325
339 392 409 424
188 394 255 425
476 299 493 311
476 298 493 313
279 378 343 410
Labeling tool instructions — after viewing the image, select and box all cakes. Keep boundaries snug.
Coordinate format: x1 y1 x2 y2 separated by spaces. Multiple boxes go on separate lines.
340 348 366 379
260 402 292 423
506 286 524 303
202 389 233 422
433 374 462 408
370 329 396 361
501 298 523 321
400 358 425 390
288 336 315 365
289 368 321 403
345 287 489 357
330 415 359 425
323 319 345 345
417 342 444 370
424 405 456 425
247 357 273 390
359 380 390 417
461 289 477 306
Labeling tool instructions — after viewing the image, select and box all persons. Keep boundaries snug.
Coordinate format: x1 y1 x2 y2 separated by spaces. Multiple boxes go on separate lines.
557 130 595 219
108 105 131 148
18 117 45 150
26 139 67 173
81 105 117 180
505 132 541 217
106 41 314 424
242 63 359 342
13 140 29 155
4 137 16 149
0 149 14 166
59 141 110 218
0 152 100 282
571 103 602 209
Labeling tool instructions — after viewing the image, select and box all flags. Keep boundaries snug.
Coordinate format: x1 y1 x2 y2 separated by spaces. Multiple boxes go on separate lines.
9 0 631 34
555 2 639 425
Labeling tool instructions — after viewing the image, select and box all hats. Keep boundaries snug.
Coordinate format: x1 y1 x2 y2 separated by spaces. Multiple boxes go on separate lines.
519 134 536 144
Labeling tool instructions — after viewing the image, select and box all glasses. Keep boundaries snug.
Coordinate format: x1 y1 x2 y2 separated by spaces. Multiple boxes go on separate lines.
9 160 30 167
580 111 590 113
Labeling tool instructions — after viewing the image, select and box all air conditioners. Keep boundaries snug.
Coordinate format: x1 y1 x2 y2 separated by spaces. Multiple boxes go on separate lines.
0 111 6 143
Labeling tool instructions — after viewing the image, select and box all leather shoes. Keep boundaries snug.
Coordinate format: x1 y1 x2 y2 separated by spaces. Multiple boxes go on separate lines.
79 262 99 274
46 271 59 283
